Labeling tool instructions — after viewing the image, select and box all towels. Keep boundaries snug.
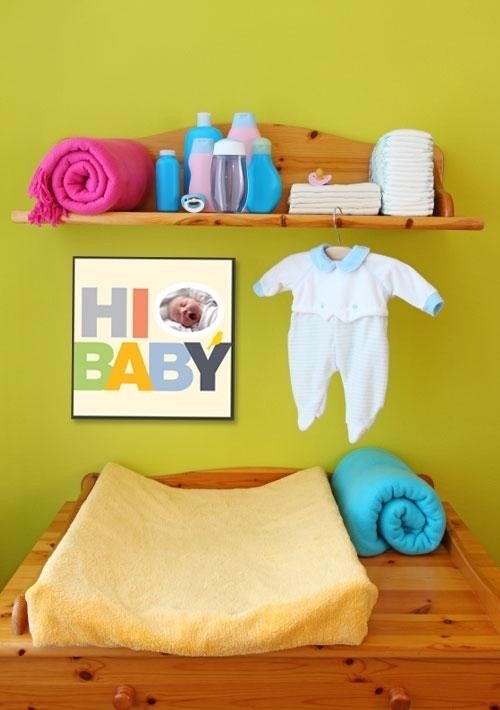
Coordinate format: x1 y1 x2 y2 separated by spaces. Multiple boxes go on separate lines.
288 180 379 220
23 464 382 656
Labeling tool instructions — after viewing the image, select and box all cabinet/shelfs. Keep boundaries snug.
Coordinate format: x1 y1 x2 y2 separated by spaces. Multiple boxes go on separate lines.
1 469 500 708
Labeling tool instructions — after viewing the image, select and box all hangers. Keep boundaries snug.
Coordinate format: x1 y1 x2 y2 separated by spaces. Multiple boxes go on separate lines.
323 203 363 265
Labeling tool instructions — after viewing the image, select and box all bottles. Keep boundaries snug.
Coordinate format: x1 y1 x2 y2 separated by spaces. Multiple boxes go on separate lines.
154 112 283 214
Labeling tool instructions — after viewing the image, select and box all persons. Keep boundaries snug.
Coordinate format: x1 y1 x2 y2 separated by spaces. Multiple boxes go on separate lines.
167 295 218 331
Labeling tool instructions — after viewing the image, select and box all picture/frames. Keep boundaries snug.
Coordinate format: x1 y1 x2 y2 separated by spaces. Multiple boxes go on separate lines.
73 255 235 420
